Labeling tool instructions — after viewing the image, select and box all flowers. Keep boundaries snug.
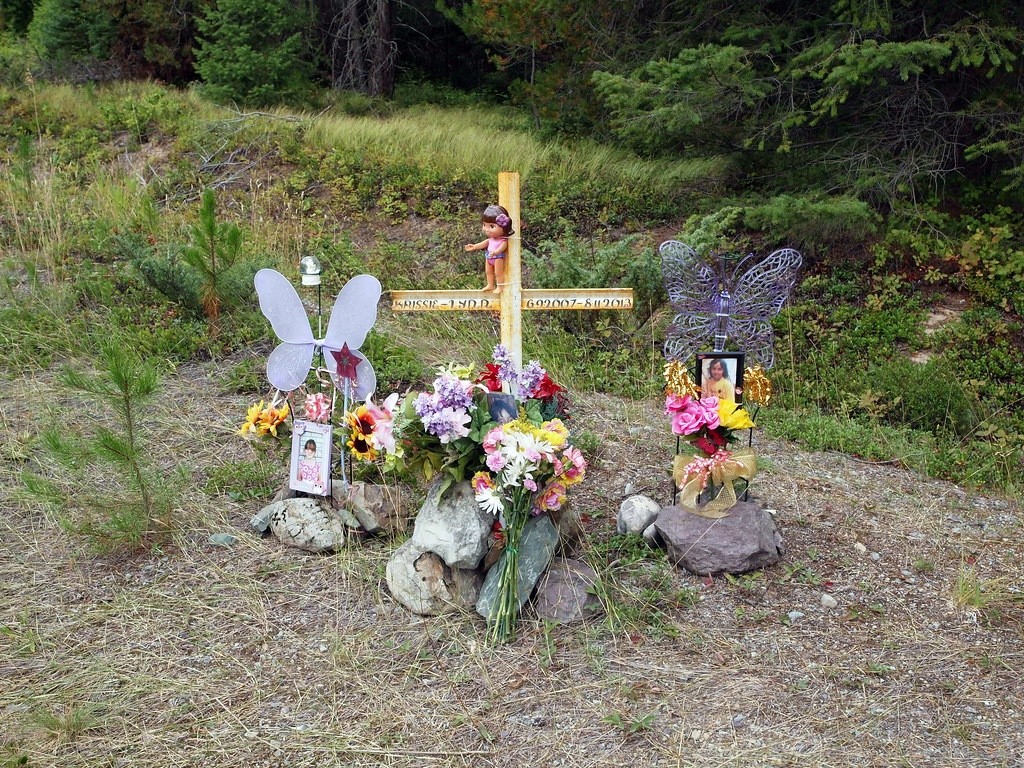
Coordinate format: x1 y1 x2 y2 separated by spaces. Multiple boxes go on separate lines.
240 344 588 644
667 394 755 501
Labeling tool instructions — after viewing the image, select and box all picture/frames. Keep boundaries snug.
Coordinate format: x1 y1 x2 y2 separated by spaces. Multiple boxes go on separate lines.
289 420 332 496
695 352 745 410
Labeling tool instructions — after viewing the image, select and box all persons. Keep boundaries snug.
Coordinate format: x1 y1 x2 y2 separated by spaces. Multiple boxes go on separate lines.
298 440 320 481
701 359 733 402
465 205 515 293
